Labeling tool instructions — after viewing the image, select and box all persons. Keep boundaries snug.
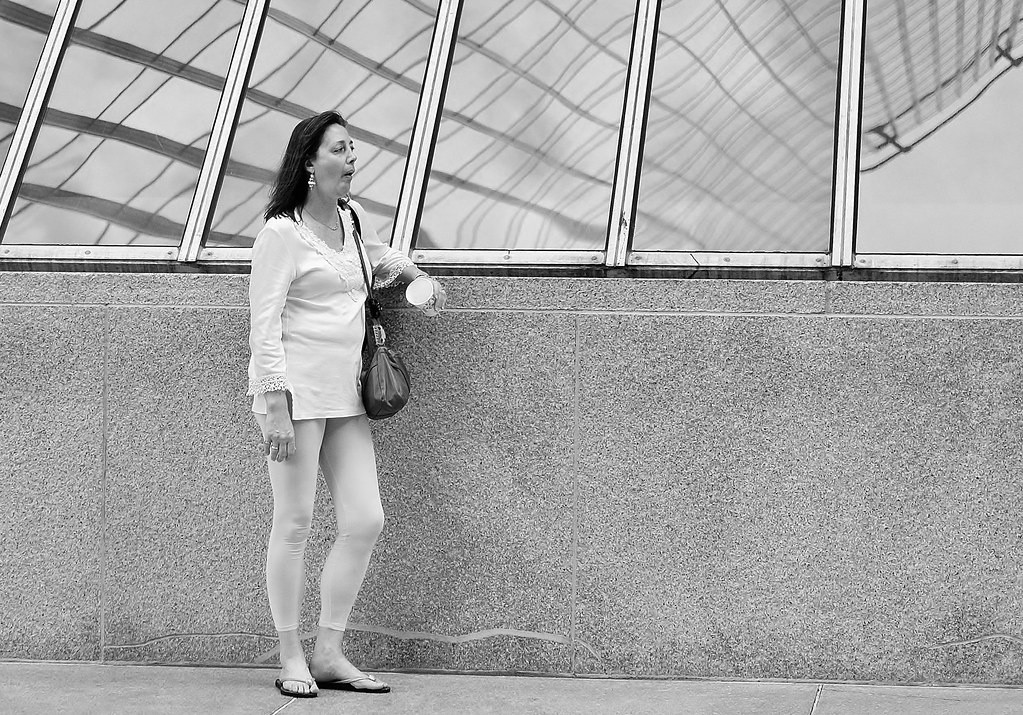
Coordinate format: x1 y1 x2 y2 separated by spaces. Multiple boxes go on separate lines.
246 109 446 698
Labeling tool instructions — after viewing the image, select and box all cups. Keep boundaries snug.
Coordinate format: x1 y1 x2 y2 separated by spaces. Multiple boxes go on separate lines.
406 279 440 317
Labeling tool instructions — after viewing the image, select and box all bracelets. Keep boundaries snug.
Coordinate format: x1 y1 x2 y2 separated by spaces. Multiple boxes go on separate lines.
414 274 431 280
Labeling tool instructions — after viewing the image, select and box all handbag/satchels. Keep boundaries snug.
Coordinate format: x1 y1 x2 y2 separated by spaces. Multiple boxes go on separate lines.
360 346 411 420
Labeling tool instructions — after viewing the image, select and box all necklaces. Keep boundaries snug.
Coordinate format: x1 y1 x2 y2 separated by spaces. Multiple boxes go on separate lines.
304 204 339 230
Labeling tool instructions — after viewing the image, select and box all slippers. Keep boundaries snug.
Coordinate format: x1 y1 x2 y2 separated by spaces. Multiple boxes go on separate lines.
275 678 317 698
316 672 390 693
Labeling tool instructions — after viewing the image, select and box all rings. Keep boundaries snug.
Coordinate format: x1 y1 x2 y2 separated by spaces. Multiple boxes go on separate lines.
441 289 444 290
264 442 271 445
271 446 278 450
